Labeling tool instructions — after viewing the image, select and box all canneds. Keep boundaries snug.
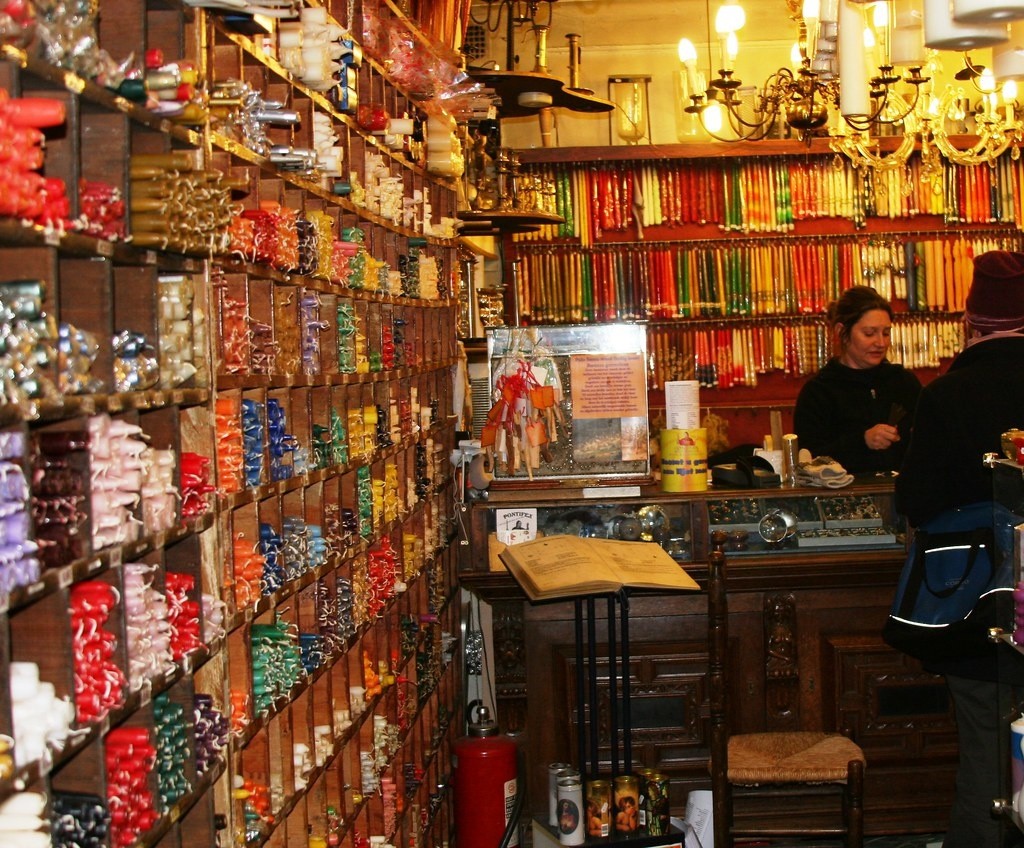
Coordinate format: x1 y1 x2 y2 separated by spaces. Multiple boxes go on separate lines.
637 768 671 837
548 762 585 847
586 780 613 838
613 776 640 834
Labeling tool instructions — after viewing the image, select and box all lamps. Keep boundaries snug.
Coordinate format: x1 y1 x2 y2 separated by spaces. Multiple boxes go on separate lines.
676 1 1024 198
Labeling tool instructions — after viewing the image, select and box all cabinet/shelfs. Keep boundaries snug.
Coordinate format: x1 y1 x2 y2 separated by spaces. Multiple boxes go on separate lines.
0 0 467 848
983 451 1024 848
501 132 1024 409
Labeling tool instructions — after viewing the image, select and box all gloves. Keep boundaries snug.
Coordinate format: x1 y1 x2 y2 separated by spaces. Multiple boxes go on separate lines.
794 448 854 488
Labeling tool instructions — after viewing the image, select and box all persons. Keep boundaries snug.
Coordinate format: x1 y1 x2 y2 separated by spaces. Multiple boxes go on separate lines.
877 250 1024 848
792 285 923 474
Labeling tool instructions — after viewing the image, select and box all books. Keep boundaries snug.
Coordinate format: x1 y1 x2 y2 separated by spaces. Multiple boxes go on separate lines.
497 533 703 606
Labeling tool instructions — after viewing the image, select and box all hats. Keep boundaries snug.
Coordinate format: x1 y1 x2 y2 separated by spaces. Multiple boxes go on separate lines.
964 250 1024 333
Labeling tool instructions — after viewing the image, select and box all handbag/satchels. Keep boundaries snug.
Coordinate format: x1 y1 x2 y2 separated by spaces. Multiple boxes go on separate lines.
881 500 1024 661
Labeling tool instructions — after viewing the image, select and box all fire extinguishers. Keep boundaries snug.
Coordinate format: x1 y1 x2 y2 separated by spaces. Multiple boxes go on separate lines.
453 699 526 848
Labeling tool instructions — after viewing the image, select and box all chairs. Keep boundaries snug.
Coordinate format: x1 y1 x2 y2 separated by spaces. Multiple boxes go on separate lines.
707 529 867 848
